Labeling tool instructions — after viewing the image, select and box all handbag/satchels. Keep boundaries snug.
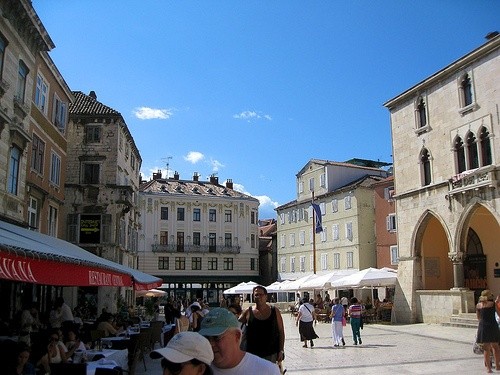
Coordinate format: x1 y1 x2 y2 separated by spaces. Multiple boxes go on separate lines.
473 342 484 355
341 307 346 327
238 306 252 351
312 314 315 321
359 305 363 329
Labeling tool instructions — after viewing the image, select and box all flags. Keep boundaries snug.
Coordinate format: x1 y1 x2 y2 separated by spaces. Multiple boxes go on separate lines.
311 202 323 234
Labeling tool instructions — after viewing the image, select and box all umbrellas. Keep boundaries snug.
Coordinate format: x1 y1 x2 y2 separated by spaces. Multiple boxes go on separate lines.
136 289 167 297
223 267 398 307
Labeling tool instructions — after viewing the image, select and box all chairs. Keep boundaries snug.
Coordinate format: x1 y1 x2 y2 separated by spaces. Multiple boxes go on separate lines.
314 306 393 325
48 320 165 375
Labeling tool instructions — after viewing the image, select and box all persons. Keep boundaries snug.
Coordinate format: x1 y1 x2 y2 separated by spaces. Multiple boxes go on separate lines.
296 297 319 348
198 307 283 375
238 286 285 375
294 292 393 324
0 286 211 375
149 331 214 375
472 289 500 373
329 297 345 347
348 297 363 346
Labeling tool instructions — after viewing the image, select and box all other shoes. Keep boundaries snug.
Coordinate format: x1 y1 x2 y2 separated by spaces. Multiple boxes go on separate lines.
303 345 307 348
334 344 339 347
342 338 345 346
353 342 357 345
358 338 362 344
310 341 314 347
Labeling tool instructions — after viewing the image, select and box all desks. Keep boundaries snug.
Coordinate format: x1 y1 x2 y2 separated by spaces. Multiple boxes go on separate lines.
87 359 118 375
74 349 129 370
102 336 130 347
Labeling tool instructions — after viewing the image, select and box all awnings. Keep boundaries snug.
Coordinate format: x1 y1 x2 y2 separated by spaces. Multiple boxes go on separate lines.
0 220 162 291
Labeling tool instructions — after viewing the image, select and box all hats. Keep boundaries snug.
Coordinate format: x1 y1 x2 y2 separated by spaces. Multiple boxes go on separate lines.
479 290 494 301
185 302 202 317
150 331 214 366
199 307 239 336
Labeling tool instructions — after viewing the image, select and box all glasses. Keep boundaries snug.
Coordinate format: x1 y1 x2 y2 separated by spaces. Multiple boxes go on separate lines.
203 329 234 343
161 358 192 375
50 337 59 342
